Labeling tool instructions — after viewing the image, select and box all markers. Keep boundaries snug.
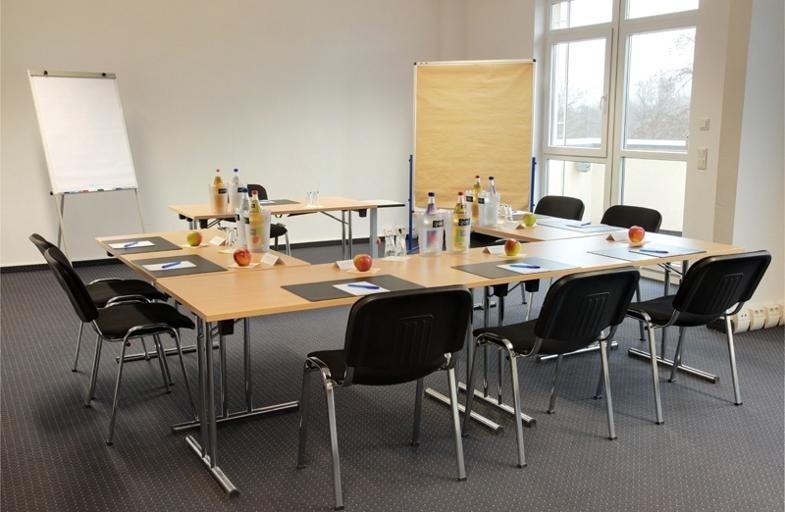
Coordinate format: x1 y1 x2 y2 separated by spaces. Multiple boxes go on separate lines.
116 188 122 190
83 190 88 192
97 189 104 191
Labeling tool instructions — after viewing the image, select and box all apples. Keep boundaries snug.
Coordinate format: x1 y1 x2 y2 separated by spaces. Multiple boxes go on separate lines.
505 239 521 256
233 248 251 266
354 255 372 272
187 231 202 246
629 226 645 243
523 213 536 226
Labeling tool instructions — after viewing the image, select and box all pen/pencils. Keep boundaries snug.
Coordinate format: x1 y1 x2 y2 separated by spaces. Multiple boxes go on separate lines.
640 249 668 253
510 264 540 268
348 284 380 289
581 223 590 226
162 261 180 268
124 242 137 247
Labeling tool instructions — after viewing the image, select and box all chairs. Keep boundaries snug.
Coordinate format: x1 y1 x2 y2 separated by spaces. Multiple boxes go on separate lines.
42 241 204 444
460 255 643 471
596 239 776 436
594 204 667 343
503 192 589 321
288 279 473 511
27 223 176 405
226 179 295 258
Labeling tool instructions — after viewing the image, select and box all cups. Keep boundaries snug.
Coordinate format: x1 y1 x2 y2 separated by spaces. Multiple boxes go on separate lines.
413 211 446 257
233 208 271 253
304 190 320 206
383 225 407 259
230 184 247 213
444 211 472 253
207 183 229 214
466 188 486 222
500 201 513 223
478 192 501 227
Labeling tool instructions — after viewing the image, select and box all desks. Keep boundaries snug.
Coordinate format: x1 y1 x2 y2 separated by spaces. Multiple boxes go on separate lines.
427 229 754 430
151 254 504 498
430 199 626 335
167 193 408 260
89 224 316 434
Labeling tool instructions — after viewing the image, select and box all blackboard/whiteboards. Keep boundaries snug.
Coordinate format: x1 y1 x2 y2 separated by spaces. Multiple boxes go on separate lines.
412 58 538 215
27 69 139 195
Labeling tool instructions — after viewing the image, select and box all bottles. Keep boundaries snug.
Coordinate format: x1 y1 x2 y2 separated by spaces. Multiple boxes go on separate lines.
212 168 225 211
451 191 468 252
424 192 439 248
472 175 482 220
486 176 497 224
231 169 241 186
248 190 264 248
240 188 250 212
